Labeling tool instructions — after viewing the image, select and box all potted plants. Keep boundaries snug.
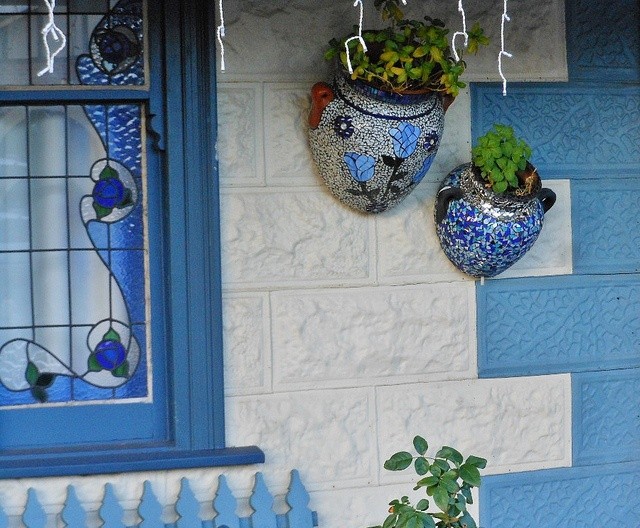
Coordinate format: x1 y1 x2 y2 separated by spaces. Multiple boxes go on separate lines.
308 1 491 214
433 126 556 277
